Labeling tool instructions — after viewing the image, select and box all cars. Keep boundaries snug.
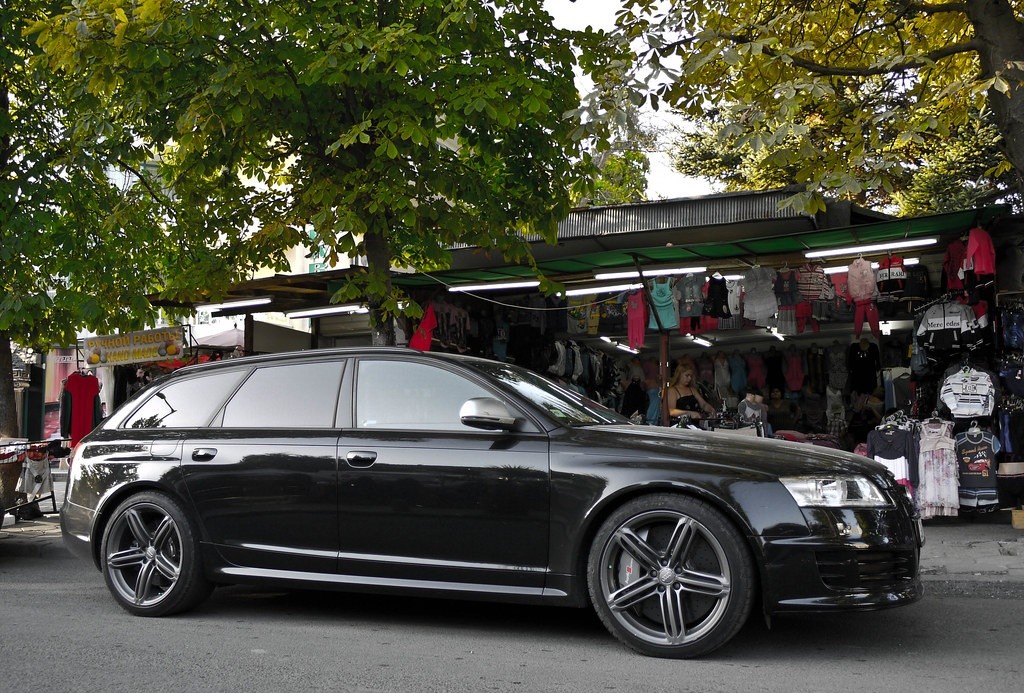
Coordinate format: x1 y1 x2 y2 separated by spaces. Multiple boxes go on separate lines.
59 347 927 659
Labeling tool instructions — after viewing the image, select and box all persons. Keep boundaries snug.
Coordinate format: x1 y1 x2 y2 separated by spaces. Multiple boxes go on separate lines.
667 362 716 426
621 338 881 434
